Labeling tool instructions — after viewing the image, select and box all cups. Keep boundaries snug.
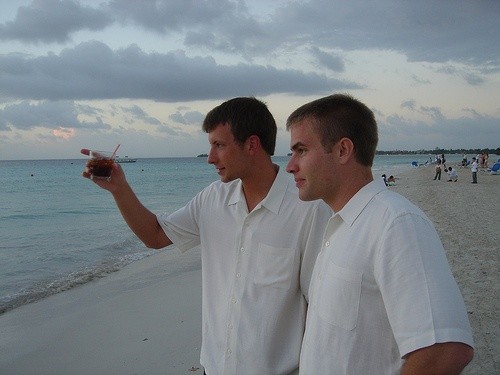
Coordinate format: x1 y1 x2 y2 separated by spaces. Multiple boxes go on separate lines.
90 150 116 182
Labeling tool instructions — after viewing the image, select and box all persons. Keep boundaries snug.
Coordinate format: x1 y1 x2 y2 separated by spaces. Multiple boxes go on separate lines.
285 93 474 375
78 97 333 375
382 150 500 186
